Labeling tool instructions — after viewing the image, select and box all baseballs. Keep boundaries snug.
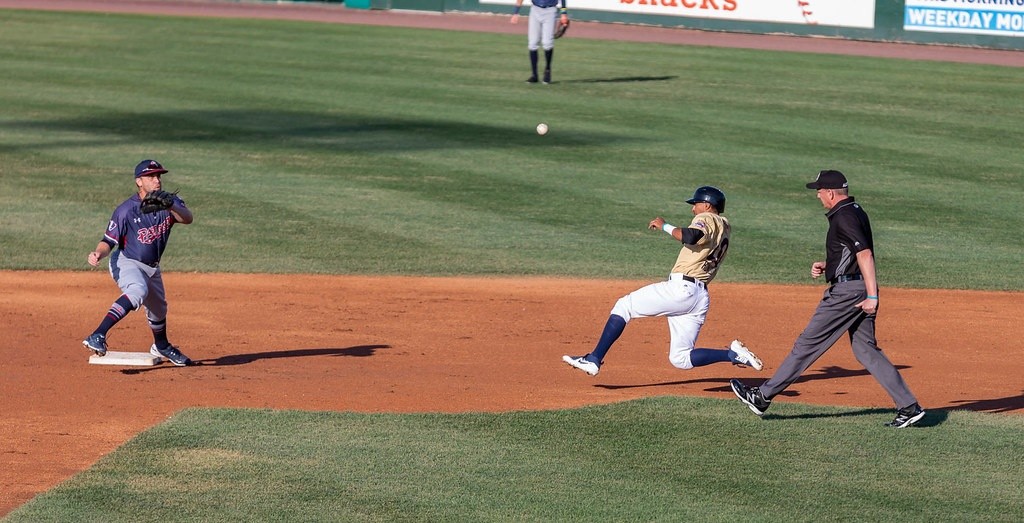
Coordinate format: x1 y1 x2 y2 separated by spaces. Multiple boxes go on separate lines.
537 123 548 135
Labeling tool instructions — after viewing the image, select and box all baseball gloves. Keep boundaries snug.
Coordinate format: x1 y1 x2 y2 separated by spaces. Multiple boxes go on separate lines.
140 187 181 216
554 20 569 39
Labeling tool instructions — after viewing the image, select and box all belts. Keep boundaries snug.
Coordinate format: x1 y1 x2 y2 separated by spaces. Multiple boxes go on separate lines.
533 3 554 8
141 261 157 267
831 274 860 285
670 275 707 290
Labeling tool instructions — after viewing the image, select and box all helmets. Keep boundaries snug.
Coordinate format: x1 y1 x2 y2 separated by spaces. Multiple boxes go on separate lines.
685 186 726 213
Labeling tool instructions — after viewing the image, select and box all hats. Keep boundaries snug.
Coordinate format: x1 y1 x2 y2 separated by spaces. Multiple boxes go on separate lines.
806 169 848 189
135 160 169 178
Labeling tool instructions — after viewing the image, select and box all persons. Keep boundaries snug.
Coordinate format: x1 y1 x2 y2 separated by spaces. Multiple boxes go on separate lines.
730 170 925 428
83 159 193 366
563 187 763 377
511 0 568 84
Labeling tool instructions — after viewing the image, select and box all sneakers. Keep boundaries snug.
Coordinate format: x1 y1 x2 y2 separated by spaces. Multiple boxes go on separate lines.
563 354 604 377
884 403 926 429
730 379 771 416
82 333 108 357
149 344 192 367
729 339 764 371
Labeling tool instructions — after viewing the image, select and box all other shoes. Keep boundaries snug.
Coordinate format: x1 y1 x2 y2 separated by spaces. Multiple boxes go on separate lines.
542 70 551 84
526 74 538 83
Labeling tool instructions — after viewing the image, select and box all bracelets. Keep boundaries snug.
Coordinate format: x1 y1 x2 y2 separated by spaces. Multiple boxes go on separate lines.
867 295 879 299
